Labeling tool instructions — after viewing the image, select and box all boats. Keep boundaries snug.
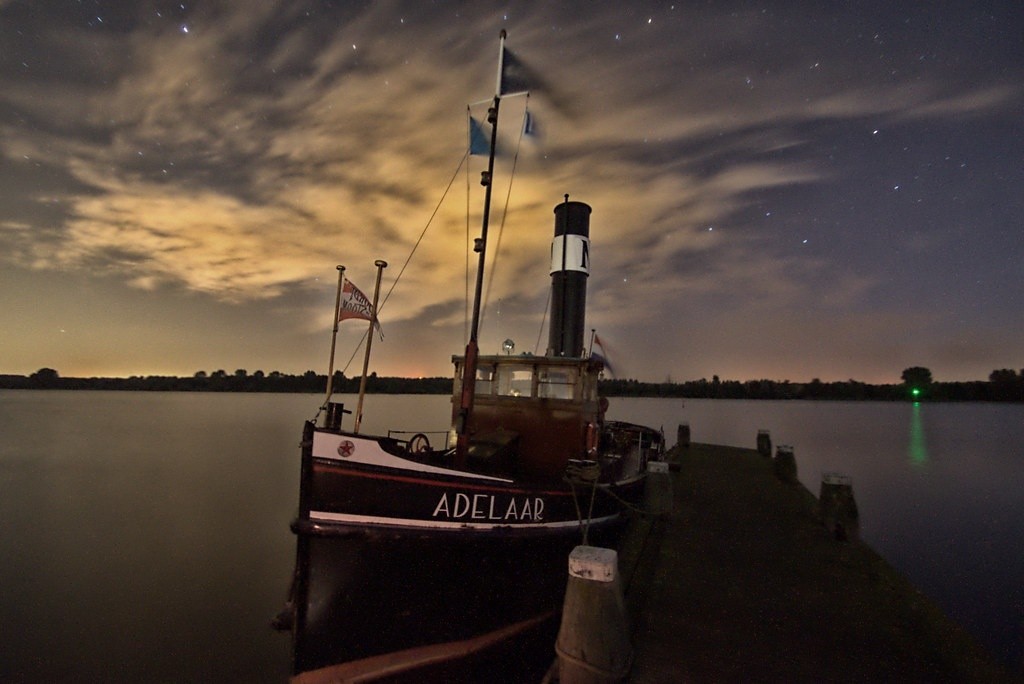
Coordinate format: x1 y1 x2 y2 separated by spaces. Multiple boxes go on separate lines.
281 26 677 683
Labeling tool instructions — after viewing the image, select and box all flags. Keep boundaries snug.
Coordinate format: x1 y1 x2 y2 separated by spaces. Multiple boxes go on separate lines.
338 271 385 343
499 48 557 97
469 117 517 160
524 112 547 140
591 335 622 377
